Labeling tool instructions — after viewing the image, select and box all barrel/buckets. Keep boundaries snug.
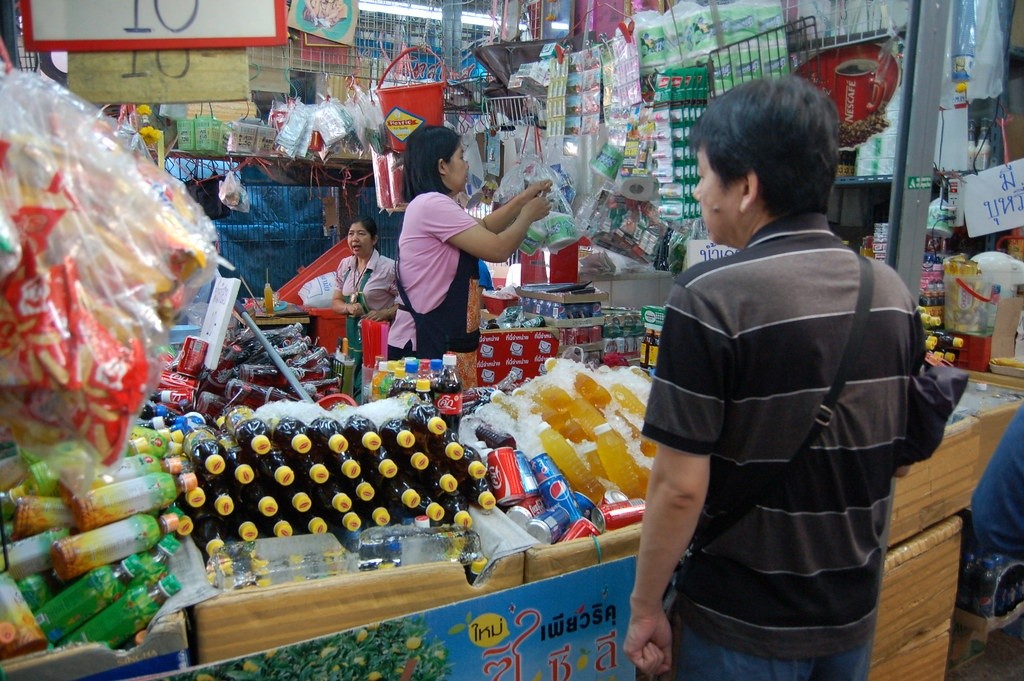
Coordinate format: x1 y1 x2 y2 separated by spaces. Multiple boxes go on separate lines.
944 274 992 332
373 46 446 153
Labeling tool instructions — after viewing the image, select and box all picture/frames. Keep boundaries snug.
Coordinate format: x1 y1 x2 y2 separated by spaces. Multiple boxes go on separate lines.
541 0 576 42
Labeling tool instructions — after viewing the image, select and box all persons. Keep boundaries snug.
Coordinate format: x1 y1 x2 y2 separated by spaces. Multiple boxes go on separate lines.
388 125 553 362
972 405 1024 564
479 260 495 309
623 78 929 681
332 219 404 405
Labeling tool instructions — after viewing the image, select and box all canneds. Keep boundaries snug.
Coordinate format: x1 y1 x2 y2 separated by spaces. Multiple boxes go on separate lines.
487 446 647 545
517 296 601 366
601 313 644 359
150 335 210 415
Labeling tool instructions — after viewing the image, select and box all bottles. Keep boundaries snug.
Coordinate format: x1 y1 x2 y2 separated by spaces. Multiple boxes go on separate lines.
0 319 659 660
968 118 998 171
264 283 273 314
918 272 963 362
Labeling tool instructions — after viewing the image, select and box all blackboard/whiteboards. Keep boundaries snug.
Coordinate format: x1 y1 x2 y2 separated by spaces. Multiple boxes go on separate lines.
19 0 287 52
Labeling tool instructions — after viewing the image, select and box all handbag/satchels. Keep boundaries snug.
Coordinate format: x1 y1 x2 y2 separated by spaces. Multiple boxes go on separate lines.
636 547 698 681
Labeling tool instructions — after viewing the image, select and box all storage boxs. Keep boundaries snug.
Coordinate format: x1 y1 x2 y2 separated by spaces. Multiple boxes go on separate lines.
0 525 645 681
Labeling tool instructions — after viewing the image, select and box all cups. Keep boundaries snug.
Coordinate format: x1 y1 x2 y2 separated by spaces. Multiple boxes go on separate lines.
835 58 886 126
